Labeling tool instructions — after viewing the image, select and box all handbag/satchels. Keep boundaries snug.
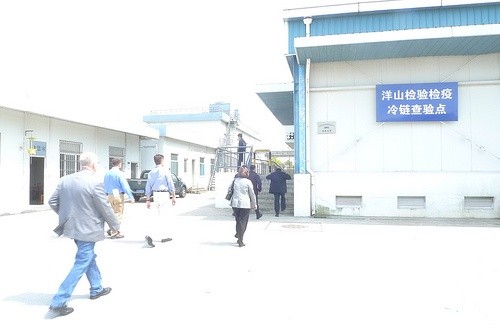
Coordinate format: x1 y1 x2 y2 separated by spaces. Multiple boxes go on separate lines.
225 178 236 200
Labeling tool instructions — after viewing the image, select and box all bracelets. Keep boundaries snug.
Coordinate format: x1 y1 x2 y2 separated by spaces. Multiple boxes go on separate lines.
146 199 150 201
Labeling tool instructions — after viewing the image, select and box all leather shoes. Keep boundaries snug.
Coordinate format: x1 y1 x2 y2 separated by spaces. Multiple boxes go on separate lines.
50 305 74 315
146 236 155 246
162 238 172 242
90 287 111 299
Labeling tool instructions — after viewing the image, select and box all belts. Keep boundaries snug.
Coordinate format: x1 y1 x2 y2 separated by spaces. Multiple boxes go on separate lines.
153 190 169 192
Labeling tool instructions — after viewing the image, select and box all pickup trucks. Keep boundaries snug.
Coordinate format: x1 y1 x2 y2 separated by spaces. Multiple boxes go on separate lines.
127 169 186 201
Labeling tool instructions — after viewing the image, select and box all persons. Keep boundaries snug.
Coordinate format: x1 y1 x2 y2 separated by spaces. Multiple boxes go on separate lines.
230 164 263 248
102 157 136 240
144 153 178 247
266 168 291 217
236 133 246 172
47 151 121 315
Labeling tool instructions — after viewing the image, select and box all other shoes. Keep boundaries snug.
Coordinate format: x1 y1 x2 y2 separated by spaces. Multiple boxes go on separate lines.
275 215 278 217
111 231 124 239
235 234 238 238
107 229 112 236
257 214 262 219
281 208 285 211
239 243 245 246
